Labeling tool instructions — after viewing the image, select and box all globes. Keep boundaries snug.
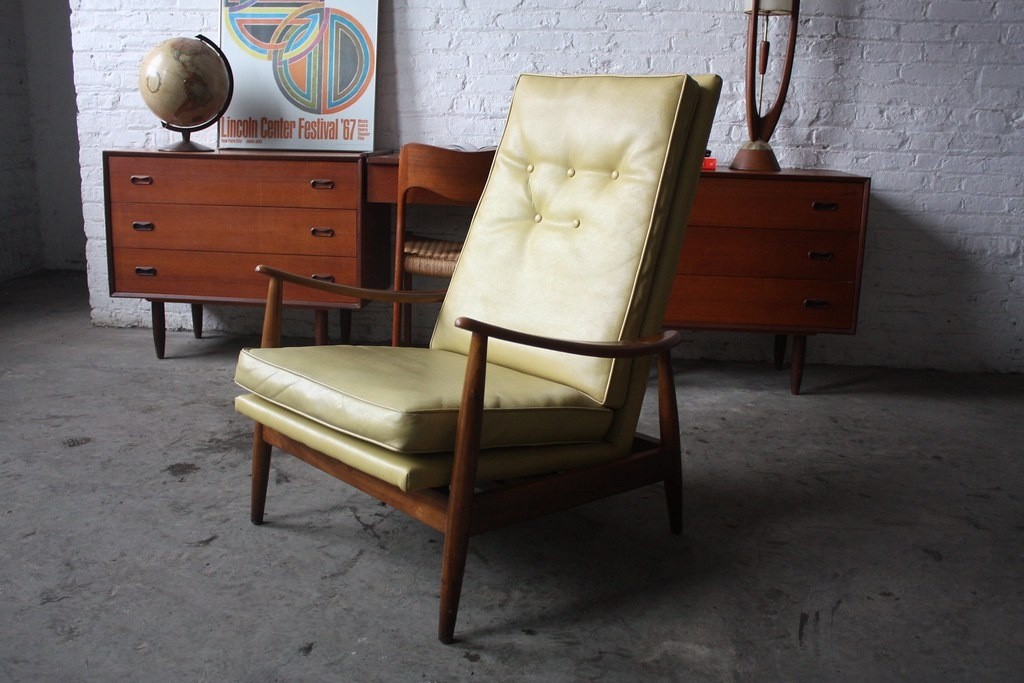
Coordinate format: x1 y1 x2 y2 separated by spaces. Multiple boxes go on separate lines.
139 34 234 152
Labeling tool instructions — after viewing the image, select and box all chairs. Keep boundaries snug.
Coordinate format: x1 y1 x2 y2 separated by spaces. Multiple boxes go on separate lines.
391 144 498 347
234 73 723 641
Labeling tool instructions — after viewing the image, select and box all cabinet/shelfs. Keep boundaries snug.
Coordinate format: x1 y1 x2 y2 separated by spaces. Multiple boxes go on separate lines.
103 149 367 357
366 153 870 393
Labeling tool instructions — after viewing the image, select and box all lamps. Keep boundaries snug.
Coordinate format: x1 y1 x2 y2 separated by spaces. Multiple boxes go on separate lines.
729 0 800 171
